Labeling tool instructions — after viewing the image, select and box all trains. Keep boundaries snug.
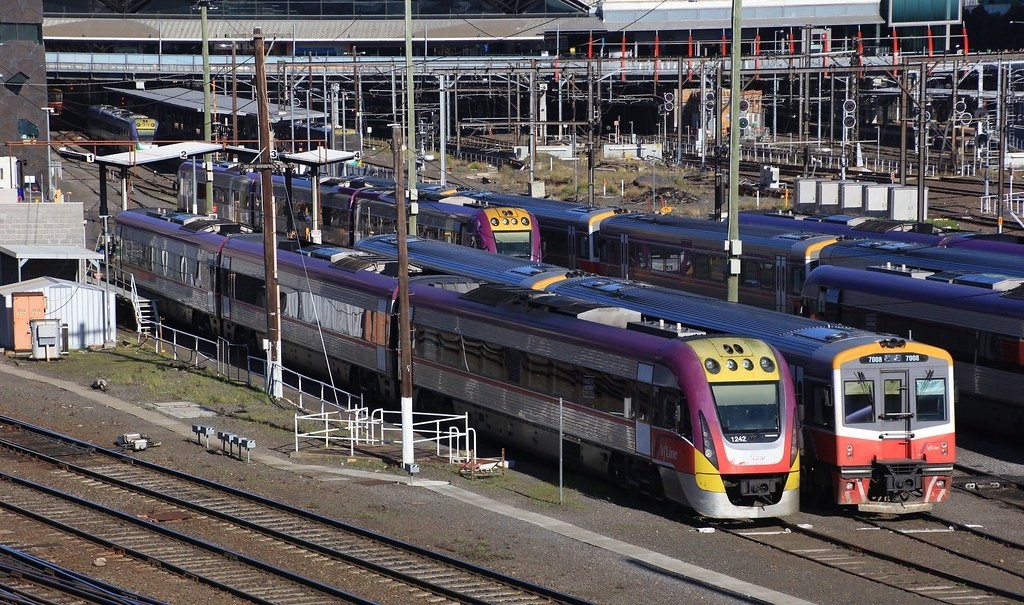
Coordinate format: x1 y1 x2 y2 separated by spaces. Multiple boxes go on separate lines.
85 105 159 154
110 207 805 526
356 233 957 514
172 157 1023 453
48 87 65 116
